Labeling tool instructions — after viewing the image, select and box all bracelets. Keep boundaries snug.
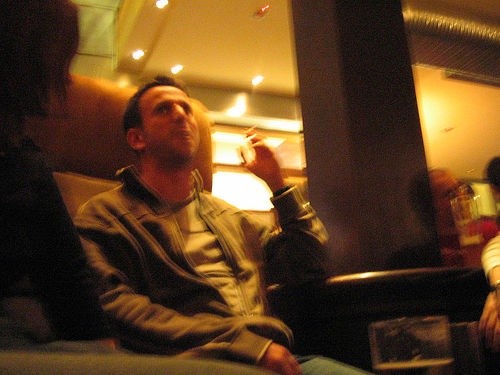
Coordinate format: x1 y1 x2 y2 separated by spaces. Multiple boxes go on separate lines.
489 280 500 294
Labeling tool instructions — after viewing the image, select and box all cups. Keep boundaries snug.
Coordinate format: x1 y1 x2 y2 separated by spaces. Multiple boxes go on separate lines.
450 195 482 247
366 316 453 375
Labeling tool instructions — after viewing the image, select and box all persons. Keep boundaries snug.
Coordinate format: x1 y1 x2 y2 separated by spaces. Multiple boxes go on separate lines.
72 76 330 375
380 163 500 326
0 1 111 347
477 236 500 355
